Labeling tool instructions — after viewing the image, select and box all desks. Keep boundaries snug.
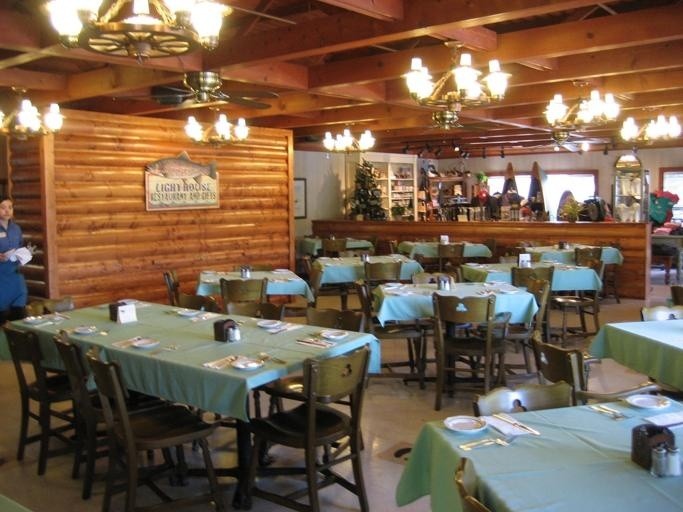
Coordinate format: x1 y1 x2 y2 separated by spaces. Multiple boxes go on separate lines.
650 233 683 284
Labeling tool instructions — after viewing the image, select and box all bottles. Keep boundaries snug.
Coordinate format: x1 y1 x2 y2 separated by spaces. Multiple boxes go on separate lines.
234 327 241 342
228 327 235 342
448 206 487 221
650 449 665 477
501 205 511 221
511 204 520 220
667 446 681 476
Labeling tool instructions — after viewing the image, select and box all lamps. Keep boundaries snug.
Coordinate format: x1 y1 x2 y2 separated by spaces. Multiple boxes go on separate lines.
322 127 377 151
46 0 230 59
401 40 512 132
184 107 250 144
0 86 64 140
401 145 507 159
576 144 637 154
620 107 681 148
541 81 620 144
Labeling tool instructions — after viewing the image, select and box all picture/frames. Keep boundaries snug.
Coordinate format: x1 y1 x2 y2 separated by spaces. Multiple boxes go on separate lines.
293 178 307 219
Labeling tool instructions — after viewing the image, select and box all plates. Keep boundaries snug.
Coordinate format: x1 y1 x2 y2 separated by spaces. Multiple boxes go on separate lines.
176 308 200 316
23 316 45 324
203 270 214 274
117 299 138 304
275 268 288 271
257 320 280 328
129 338 160 348
477 290 494 295
384 283 401 286
232 358 262 371
201 278 218 283
483 281 506 286
324 263 335 266
319 257 329 259
386 289 408 296
322 327 348 339
559 266 573 270
444 415 487 431
498 288 518 293
575 266 588 269
272 268 288 273
273 278 289 282
626 395 672 408
486 269 502 272
74 327 96 334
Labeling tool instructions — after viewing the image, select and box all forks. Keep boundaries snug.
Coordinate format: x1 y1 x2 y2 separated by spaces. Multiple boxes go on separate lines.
270 328 287 334
589 405 623 421
34 320 64 329
458 434 517 451
258 350 285 364
81 328 112 336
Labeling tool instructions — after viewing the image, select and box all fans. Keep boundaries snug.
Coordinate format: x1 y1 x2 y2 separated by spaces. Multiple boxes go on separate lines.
111 73 280 109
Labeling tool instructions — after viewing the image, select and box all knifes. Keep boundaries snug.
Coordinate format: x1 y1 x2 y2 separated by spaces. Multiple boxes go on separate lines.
492 413 540 436
599 405 635 418
297 338 325 348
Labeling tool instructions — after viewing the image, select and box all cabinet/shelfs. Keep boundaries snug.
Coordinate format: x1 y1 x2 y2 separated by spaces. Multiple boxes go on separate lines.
345 151 418 222
422 175 474 221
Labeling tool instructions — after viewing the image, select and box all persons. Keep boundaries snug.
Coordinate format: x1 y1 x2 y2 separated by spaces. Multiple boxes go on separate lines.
0 195 29 363
